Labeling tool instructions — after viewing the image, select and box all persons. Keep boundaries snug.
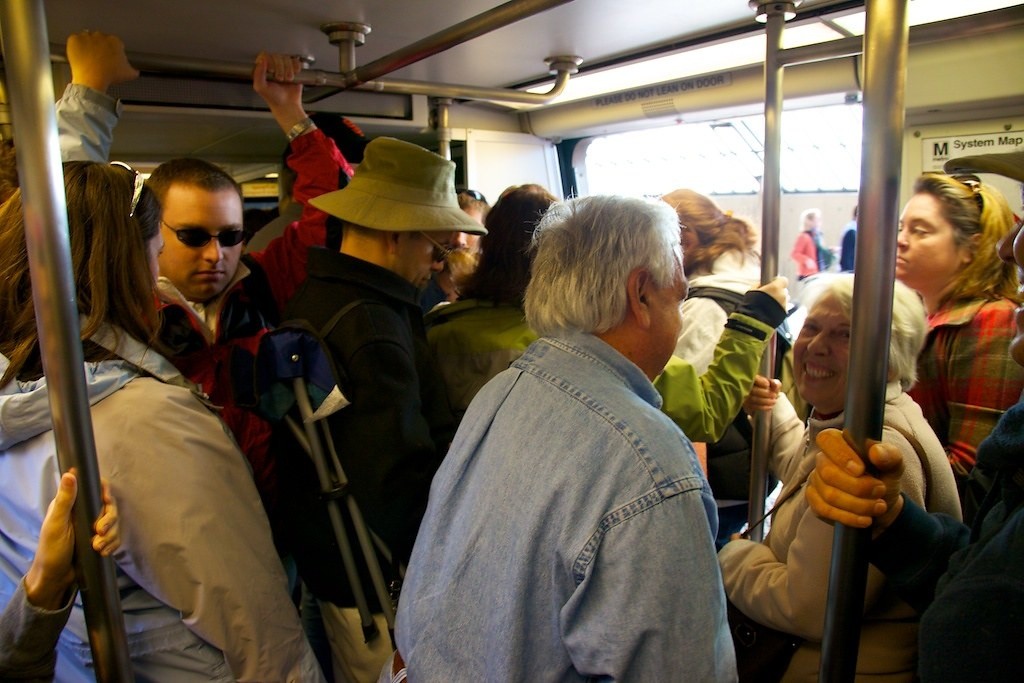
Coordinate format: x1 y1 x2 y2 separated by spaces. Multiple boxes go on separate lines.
894 171 1020 511
0 30 802 682
717 270 964 683
803 142 1024 683
1 470 125 682
839 205 858 274
791 212 823 282
394 191 740 683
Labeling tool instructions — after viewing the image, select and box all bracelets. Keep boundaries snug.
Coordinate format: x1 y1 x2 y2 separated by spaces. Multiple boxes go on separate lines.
285 117 314 143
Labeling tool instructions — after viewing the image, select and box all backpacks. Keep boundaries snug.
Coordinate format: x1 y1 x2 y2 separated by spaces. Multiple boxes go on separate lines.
804 228 833 271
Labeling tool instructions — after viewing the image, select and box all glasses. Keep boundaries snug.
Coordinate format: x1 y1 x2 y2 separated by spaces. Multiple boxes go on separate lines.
420 231 453 262
952 174 985 212
456 188 488 204
110 160 145 216
164 222 245 248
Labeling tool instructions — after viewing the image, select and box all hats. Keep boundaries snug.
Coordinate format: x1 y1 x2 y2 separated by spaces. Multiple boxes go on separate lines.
308 136 488 235
